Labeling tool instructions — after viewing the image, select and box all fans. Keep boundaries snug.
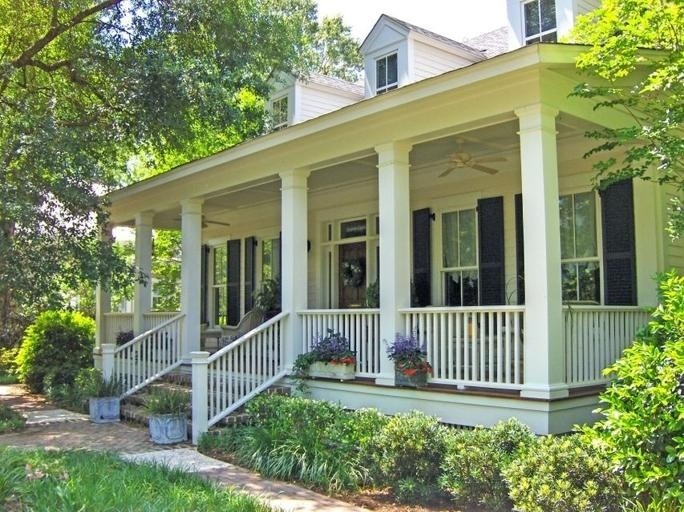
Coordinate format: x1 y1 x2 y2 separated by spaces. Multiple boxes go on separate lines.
437 138 510 179
173 212 231 229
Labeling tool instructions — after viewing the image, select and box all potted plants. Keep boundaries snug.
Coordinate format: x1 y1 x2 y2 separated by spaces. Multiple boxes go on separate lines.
86 369 126 423
142 378 192 445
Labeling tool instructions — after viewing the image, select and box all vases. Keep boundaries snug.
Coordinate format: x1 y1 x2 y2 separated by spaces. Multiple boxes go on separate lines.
310 361 355 382
396 370 427 388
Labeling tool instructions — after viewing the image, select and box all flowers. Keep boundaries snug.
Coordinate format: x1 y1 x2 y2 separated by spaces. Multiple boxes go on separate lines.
291 328 356 395
382 331 433 377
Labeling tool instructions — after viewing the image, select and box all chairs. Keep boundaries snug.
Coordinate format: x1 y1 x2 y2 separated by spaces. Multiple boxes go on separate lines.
219 306 264 351
